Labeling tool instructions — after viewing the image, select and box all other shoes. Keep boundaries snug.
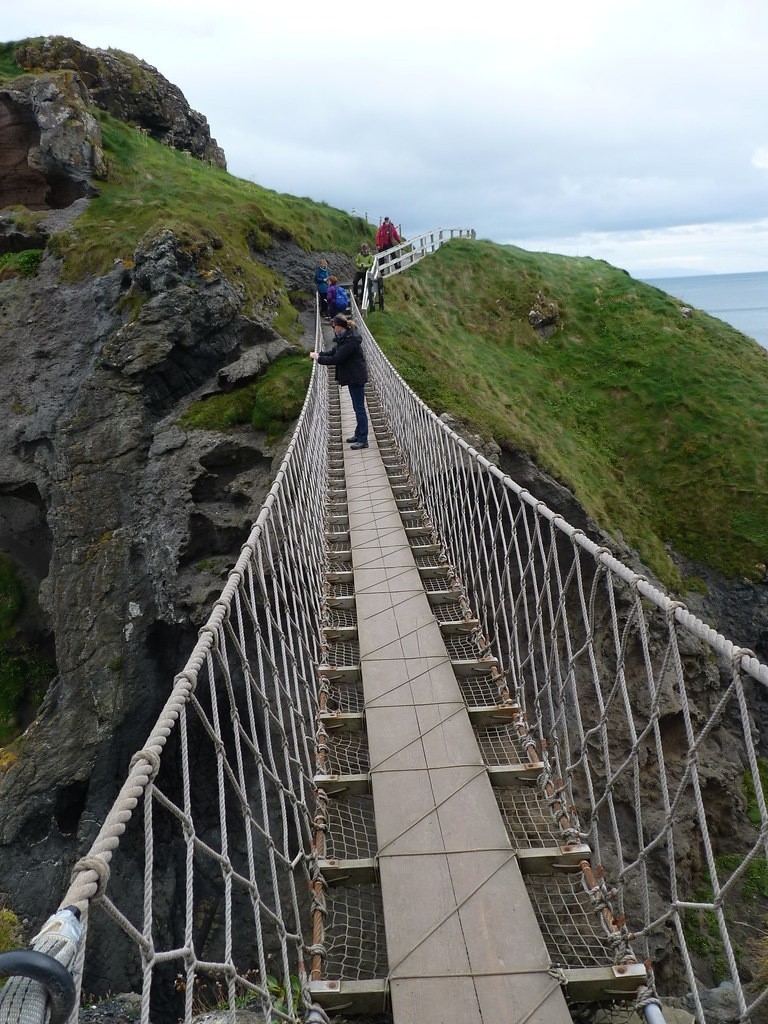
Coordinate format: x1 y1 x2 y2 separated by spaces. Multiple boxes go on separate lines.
320 311 326 317
325 312 330 317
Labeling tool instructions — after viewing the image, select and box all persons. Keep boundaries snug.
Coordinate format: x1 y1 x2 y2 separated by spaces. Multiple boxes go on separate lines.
310 314 370 451
354 243 374 301
314 259 337 318
374 216 404 279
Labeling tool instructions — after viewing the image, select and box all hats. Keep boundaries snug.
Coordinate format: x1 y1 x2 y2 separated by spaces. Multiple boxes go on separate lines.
330 316 347 326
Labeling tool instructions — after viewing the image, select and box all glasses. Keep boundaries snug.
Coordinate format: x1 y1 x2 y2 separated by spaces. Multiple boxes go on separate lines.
331 324 339 329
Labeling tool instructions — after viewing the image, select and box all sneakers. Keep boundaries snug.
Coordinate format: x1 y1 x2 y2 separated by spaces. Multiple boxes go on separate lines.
347 436 358 442
350 441 368 450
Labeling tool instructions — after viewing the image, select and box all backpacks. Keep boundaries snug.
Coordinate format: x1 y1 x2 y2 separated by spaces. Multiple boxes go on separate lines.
334 285 348 311
